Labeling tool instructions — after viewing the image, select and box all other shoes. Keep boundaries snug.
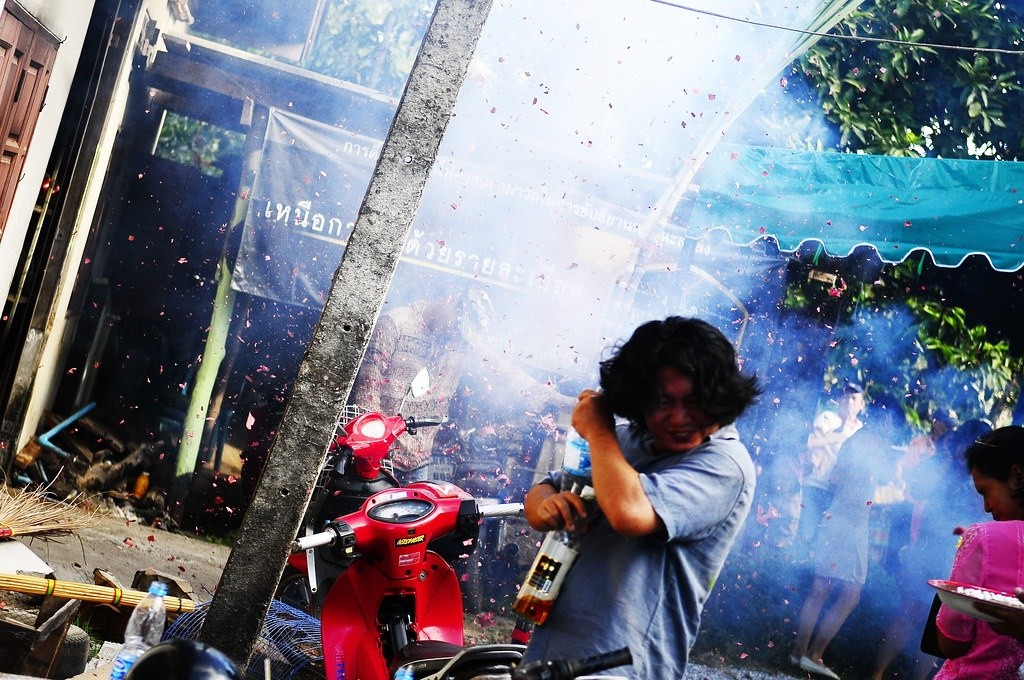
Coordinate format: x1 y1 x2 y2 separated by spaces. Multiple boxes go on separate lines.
788 654 799 665
799 655 840 680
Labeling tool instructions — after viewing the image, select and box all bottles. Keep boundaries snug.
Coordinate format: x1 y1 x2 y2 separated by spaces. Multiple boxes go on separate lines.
511 485 597 625
108 581 170 680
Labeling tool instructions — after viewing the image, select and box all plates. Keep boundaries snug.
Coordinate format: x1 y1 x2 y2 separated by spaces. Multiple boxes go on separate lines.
927 579 1024 623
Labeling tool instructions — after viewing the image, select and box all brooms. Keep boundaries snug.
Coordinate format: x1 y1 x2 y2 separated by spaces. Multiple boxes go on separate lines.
1 572 196 615
1 464 112 538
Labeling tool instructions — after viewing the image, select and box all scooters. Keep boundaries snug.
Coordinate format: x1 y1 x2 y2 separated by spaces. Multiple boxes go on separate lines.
289 365 634 680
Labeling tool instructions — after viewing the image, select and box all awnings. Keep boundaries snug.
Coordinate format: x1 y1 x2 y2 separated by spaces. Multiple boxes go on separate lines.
684 140 1024 273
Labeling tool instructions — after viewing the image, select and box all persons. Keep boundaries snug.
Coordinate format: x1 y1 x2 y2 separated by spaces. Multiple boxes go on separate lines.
706 310 1024 680
349 269 582 494
511 315 767 680
